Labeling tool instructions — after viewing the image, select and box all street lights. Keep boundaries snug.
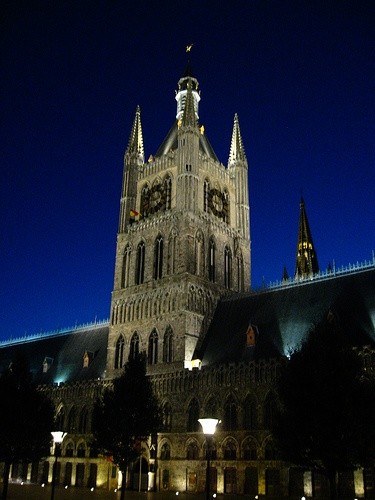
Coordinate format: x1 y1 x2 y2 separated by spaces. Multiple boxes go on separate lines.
198 416 220 500
50 431 68 500
